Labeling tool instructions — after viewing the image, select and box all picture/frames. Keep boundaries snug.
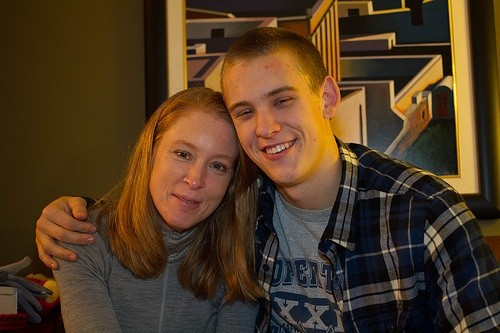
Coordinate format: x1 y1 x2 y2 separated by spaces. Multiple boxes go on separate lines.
165 0 482 197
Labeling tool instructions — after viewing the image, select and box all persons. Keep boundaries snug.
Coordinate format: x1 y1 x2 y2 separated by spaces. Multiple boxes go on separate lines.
51 88 271 333
34 27 500 333
